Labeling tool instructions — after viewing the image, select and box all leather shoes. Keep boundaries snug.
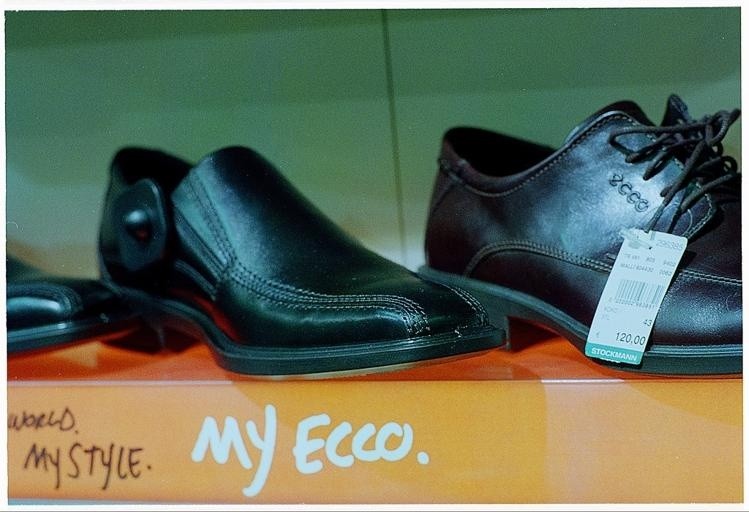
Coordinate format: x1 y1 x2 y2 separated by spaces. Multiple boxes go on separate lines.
8 256 131 353
99 145 508 377
419 94 742 377
